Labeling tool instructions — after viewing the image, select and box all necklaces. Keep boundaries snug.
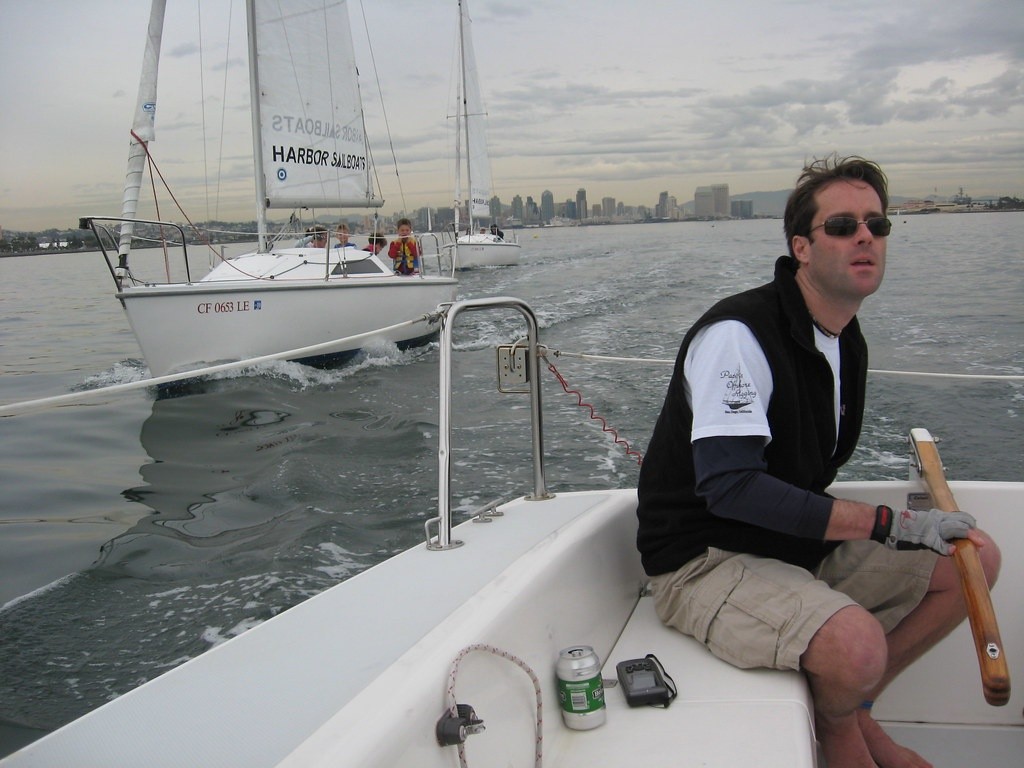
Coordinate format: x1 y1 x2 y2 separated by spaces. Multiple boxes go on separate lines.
809 310 841 339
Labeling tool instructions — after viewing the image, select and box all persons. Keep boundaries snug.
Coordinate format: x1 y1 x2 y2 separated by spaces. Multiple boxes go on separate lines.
294 225 328 248
636 150 1001 768
361 233 387 256
491 224 504 240
480 228 486 234
335 224 357 248
388 219 423 275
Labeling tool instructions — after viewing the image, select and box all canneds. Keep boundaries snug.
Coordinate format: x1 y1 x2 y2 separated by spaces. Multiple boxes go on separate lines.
556 645 606 730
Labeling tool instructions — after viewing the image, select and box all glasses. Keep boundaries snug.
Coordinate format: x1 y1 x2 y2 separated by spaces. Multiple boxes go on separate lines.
809 218 892 237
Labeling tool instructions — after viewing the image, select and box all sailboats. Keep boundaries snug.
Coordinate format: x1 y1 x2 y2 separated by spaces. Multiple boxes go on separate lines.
76 0 461 387
436 0 522 272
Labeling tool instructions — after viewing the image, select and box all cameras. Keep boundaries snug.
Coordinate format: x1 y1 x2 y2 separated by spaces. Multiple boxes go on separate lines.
616 657 670 708
